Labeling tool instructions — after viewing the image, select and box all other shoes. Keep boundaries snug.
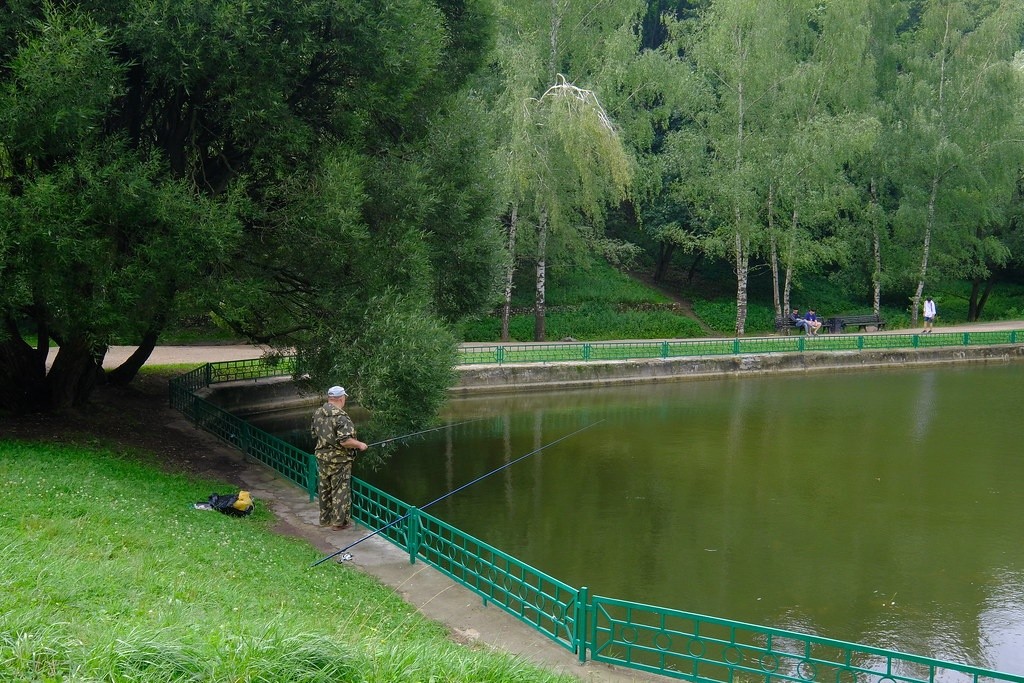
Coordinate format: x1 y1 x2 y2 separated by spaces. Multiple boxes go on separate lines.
332 521 354 531
929 330 933 333
922 330 927 333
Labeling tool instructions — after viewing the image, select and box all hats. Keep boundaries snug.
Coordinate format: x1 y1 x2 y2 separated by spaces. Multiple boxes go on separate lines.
927 296 932 301
328 386 349 397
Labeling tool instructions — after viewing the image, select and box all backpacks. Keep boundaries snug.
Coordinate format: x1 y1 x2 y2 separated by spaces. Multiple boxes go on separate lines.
208 489 254 518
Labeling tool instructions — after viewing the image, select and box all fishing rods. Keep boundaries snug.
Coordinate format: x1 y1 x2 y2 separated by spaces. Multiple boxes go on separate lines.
310 418 606 568
357 418 483 450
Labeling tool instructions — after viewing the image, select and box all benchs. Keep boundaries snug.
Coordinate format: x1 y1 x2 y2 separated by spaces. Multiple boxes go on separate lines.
841 315 886 332
782 316 833 335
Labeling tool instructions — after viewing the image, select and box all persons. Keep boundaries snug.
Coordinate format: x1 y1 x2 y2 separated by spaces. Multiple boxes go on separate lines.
790 306 810 337
922 297 936 333
804 307 822 336
311 386 368 530
